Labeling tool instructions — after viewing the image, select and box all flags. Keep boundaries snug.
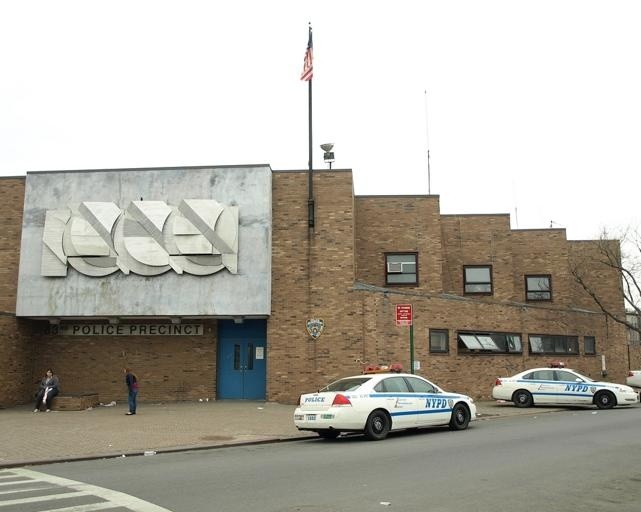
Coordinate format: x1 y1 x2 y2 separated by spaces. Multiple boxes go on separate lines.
301 26 314 81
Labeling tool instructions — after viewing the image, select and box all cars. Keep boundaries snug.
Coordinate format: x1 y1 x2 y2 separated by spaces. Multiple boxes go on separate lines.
624 369 641 388
490 359 640 411
289 361 480 442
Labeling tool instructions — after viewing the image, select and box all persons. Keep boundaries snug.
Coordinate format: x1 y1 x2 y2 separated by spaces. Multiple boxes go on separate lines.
33 367 60 412
123 365 139 415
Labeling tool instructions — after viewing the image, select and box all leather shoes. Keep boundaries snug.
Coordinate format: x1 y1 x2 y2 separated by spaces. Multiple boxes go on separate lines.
34 408 50 413
125 411 136 416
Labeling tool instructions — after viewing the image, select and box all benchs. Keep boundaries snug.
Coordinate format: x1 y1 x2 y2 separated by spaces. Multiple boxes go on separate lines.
50 392 99 411
